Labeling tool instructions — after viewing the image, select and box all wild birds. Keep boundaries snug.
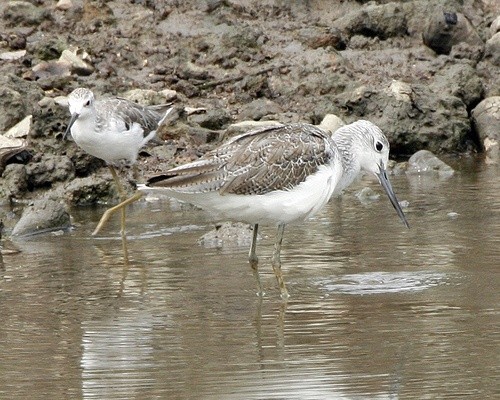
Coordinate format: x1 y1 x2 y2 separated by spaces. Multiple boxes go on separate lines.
123 112 411 300
61 86 174 233
